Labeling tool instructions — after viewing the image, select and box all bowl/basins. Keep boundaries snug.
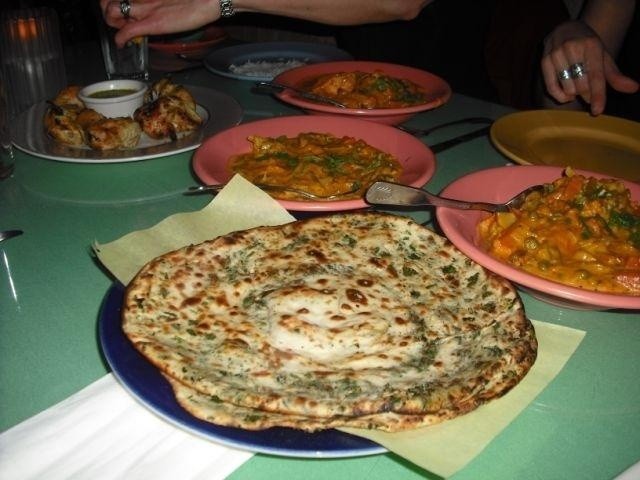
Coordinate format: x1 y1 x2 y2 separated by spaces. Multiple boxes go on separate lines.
77 78 147 120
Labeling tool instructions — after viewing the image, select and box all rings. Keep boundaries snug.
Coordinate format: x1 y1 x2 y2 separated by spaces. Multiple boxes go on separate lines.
119 0 130 19
570 62 585 80
556 69 574 79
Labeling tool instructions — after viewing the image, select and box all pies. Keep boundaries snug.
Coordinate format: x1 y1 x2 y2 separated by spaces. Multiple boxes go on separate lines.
124 209 539 434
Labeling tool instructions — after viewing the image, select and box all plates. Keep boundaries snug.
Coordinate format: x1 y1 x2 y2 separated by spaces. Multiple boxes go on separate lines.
506 290 640 420
200 41 355 87
95 273 390 458
434 165 639 317
150 24 230 53
188 112 439 217
266 60 451 126
489 107 639 182
1 85 243 163
15 154 214 210
148 51 209 76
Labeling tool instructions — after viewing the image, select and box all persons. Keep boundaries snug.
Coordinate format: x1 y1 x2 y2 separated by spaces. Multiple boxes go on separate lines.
98 1 432 49
536 0 640 120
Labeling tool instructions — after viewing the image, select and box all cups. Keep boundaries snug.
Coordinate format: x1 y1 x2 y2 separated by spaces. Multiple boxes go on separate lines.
0 2 75 114
92 17 149 84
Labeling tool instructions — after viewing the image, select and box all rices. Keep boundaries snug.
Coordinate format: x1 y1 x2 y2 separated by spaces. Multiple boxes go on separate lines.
231 62 303 76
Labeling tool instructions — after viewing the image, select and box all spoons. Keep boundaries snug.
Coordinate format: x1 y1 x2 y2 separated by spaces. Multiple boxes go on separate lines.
387 113 497 139
182 181 362 203
363 180 544 217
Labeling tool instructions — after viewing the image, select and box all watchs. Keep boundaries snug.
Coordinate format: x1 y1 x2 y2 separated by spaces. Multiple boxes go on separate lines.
218 0 235 18
